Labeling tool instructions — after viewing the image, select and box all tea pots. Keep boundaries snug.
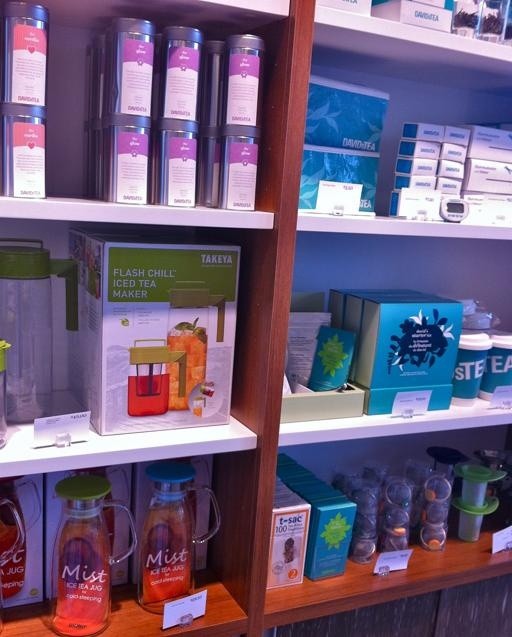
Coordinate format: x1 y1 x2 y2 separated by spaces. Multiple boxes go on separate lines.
184 457 212 568
63 462 131 556
49 475 138 635
133 461 222 615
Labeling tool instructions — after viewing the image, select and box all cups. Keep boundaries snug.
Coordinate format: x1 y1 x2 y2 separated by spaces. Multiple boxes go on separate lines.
334 454 455 566
447 330 512 408
429 445 507 544
449 0 512 46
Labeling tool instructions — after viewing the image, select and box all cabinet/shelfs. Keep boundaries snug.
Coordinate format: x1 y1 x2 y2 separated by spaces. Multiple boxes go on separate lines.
206 0 511 635
1 1 306 636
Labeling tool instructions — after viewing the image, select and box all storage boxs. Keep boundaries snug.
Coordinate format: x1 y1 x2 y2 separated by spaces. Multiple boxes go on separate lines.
295 137 381 220
64 225 248 440
302 68 390 151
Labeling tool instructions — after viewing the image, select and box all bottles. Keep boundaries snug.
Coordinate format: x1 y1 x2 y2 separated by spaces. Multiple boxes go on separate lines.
165 276 229 411
85 17 268 213
0 236 80 430
1 476 42 600
3 2 47 200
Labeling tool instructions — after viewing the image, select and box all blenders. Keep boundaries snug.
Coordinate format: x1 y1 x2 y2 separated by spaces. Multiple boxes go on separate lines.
127 338 187 417
